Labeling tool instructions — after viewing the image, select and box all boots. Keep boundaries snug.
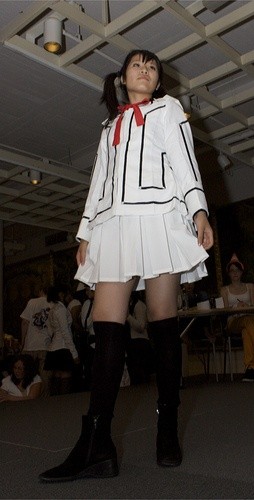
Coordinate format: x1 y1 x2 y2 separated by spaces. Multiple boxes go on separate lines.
39 320 125 481
146 315 182 466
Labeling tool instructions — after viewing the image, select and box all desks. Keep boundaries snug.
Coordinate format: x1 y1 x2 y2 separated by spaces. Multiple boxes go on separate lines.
176 306 254 383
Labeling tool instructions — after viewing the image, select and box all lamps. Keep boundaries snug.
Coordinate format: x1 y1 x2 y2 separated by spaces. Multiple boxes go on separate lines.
29 170 40 185
217 152 231 170
178 95 192 120
43 17 63 53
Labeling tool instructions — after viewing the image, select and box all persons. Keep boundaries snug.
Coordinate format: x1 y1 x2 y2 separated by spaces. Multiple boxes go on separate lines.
39 49 213 481
0 355 42 403
20 277 212 397
220 253 254 382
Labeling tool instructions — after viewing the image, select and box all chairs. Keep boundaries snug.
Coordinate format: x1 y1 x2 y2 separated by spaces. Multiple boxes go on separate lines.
180 314 244 383
